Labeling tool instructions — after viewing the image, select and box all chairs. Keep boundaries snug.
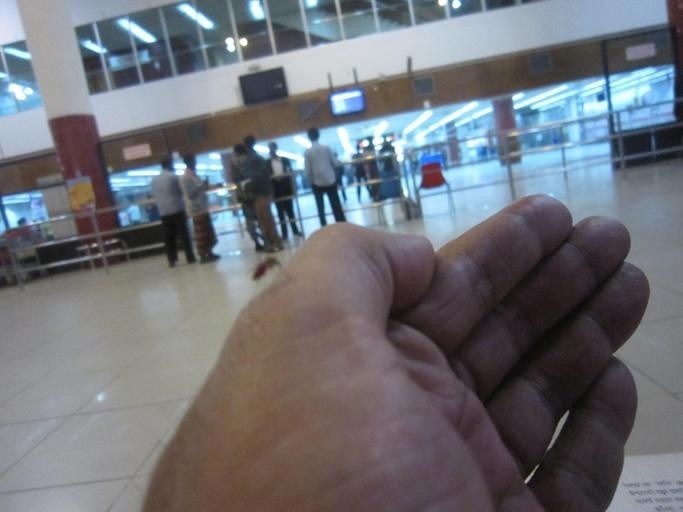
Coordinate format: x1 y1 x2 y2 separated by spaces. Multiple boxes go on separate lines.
415 161 456 217
365 170 409 225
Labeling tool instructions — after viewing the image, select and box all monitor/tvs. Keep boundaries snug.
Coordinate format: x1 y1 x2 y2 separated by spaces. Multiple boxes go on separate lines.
328 86 367 117
238 67 289 104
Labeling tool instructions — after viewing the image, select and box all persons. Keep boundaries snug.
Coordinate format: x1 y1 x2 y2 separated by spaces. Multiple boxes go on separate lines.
16 219 34 244
121 130 398 267
176 41 198 72
143 196 650 509
